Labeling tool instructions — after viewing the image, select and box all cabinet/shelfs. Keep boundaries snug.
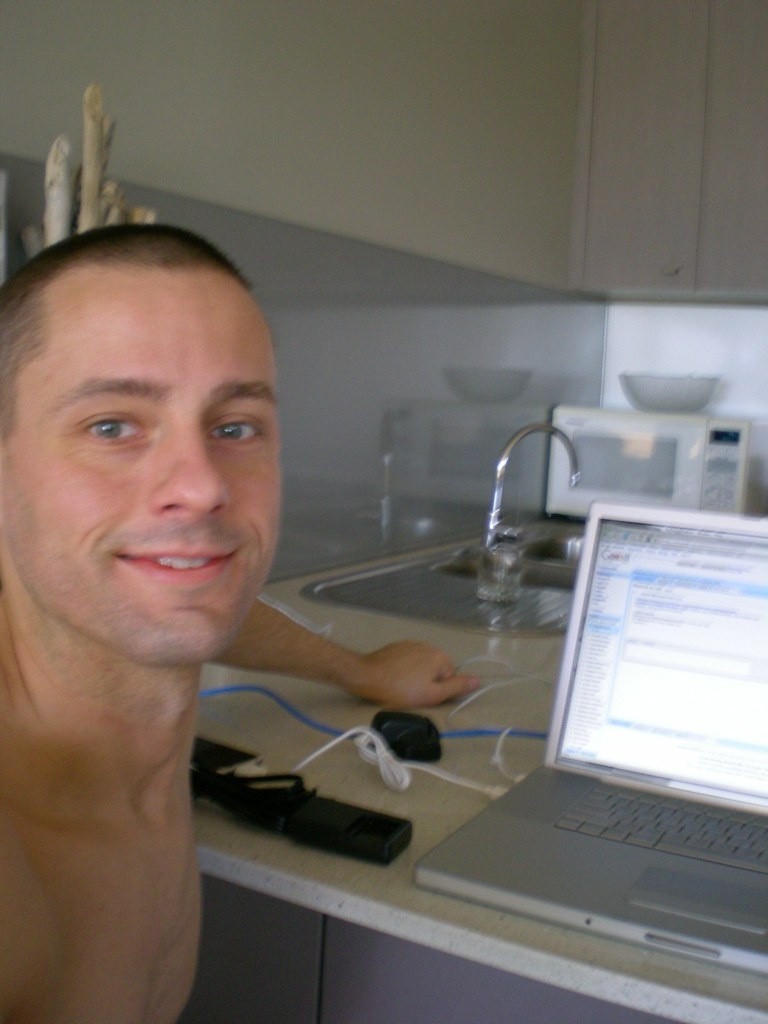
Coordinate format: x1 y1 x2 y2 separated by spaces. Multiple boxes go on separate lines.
569 0 768 302
176 871 691 1024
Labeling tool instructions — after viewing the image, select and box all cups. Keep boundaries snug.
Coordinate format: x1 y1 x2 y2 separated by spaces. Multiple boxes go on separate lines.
477 546 523 602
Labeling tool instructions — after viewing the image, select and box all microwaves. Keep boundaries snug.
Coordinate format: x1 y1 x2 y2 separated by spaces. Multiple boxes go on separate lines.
377 399 546 517
545 404 751 519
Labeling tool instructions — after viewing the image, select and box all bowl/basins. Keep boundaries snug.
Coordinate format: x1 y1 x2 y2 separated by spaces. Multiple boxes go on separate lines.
438 364 531 402
618 373 719 412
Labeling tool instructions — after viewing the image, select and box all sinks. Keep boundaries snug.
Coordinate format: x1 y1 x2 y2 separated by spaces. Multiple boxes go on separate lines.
430 552 578 601
513 532 586 568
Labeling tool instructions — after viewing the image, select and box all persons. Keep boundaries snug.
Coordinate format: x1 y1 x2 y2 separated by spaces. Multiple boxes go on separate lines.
0 224 477 1024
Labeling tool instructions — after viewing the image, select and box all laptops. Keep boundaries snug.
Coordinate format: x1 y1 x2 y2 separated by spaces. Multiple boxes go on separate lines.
415 501 768 974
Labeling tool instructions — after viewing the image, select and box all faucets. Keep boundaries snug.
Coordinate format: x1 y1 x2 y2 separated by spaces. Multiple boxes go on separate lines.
485 422 581 541
